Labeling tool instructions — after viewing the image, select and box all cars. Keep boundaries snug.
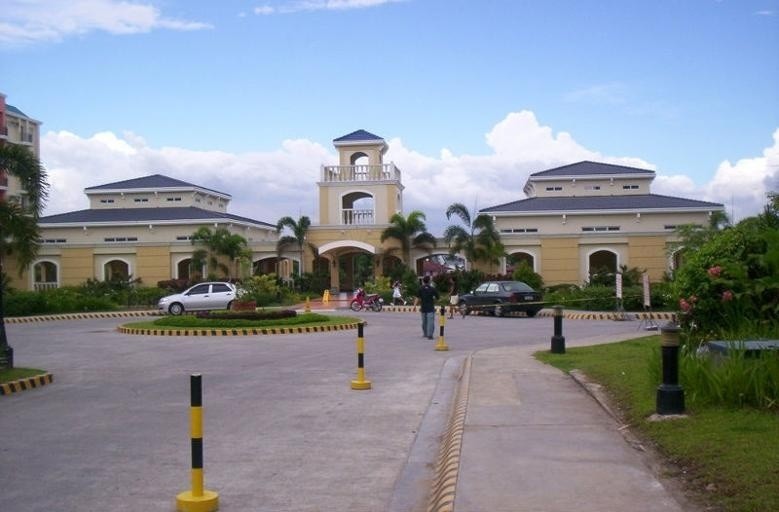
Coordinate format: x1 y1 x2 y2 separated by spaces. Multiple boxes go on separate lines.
459 280 544 317
158 281 243 316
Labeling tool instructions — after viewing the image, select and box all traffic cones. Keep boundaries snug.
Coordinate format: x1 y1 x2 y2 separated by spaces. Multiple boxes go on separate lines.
306 297 311 311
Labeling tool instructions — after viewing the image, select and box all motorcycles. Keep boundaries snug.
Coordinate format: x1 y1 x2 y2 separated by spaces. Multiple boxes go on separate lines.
350 288 384 311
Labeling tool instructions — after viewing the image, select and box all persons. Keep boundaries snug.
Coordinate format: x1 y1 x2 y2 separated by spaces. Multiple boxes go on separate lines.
448 277 467 319
413 275 439 339
389 279 407 306
